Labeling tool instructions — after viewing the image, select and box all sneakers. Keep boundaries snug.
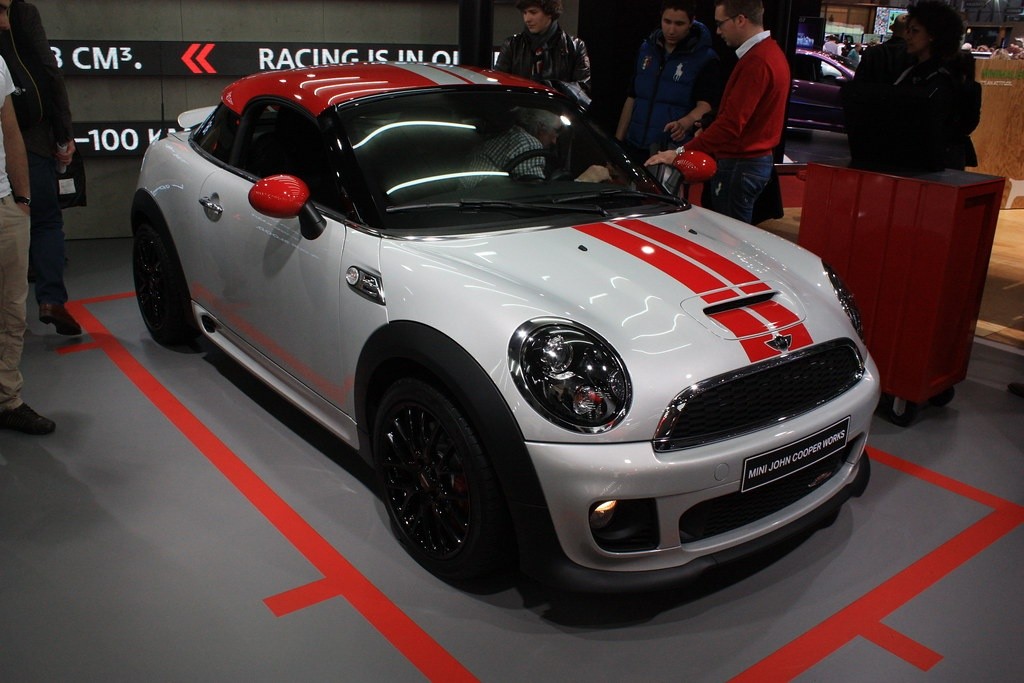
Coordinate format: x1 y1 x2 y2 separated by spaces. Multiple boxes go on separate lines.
0 402 55 435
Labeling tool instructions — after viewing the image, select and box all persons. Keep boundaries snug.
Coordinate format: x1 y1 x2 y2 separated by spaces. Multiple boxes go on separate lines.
0 0 83 433
493 0 592 96
839 0 982 171
614 0 790 224
824 36 877 70
452 106 613 202
961 36 1024 61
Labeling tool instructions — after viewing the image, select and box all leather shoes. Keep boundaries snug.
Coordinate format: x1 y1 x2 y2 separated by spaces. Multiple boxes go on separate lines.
39 304 82 335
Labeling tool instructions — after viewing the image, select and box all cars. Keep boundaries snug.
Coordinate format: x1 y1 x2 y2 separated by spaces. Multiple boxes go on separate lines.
126 63 879 597
781 48 856 131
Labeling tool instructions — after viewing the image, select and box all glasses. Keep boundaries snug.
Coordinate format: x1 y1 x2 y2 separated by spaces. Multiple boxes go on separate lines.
553 126 562 134
715 13 748 27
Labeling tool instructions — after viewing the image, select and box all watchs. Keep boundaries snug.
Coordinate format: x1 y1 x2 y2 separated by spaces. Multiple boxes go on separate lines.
15 196 32 206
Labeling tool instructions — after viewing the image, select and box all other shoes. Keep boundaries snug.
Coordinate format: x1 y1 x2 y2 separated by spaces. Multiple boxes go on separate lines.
1008 381 1024 397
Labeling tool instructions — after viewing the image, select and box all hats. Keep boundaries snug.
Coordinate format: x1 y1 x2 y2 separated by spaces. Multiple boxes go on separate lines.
889 15 908 31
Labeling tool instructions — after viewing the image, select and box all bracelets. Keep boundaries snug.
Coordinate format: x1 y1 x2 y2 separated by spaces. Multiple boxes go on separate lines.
614 137 622 145
